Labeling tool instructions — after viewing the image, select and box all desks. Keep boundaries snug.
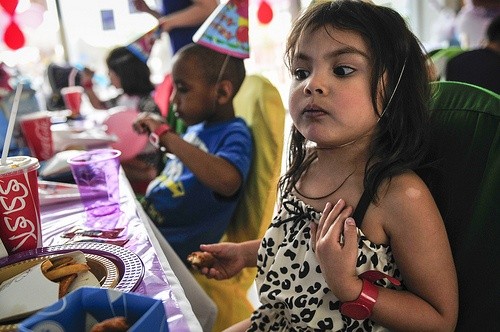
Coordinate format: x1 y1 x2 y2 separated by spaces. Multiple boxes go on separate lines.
0 109 218 332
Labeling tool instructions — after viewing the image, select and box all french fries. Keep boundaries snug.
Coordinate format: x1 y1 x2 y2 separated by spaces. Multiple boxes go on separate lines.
42 257 91 296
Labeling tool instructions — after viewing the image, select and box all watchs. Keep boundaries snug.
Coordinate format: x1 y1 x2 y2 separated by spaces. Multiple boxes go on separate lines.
150 123 173 142
339 270 401 321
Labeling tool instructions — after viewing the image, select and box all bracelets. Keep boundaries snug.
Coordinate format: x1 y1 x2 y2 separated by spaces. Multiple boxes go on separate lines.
84 82 92 88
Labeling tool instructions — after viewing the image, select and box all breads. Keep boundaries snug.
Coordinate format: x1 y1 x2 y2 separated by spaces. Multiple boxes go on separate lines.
187 251 216 268
90 317 129 332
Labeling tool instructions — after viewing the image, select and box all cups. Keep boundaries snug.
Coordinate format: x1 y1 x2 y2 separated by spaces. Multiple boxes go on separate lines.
61 86 84 118
66 149 121 220
0 156 42 255
17 111 53 162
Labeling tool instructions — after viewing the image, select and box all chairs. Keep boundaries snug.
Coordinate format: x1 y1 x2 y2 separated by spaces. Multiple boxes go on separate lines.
413 81 500 332
192 75 285 332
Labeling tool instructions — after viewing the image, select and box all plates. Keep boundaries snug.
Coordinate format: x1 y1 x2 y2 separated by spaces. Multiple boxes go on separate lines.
0 242 145 332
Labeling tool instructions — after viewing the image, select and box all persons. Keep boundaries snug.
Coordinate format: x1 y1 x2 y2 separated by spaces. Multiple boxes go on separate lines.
79 46 165 191
132 0 221 57
193 0 459 332
447 16 500 96
138 42 254 269
